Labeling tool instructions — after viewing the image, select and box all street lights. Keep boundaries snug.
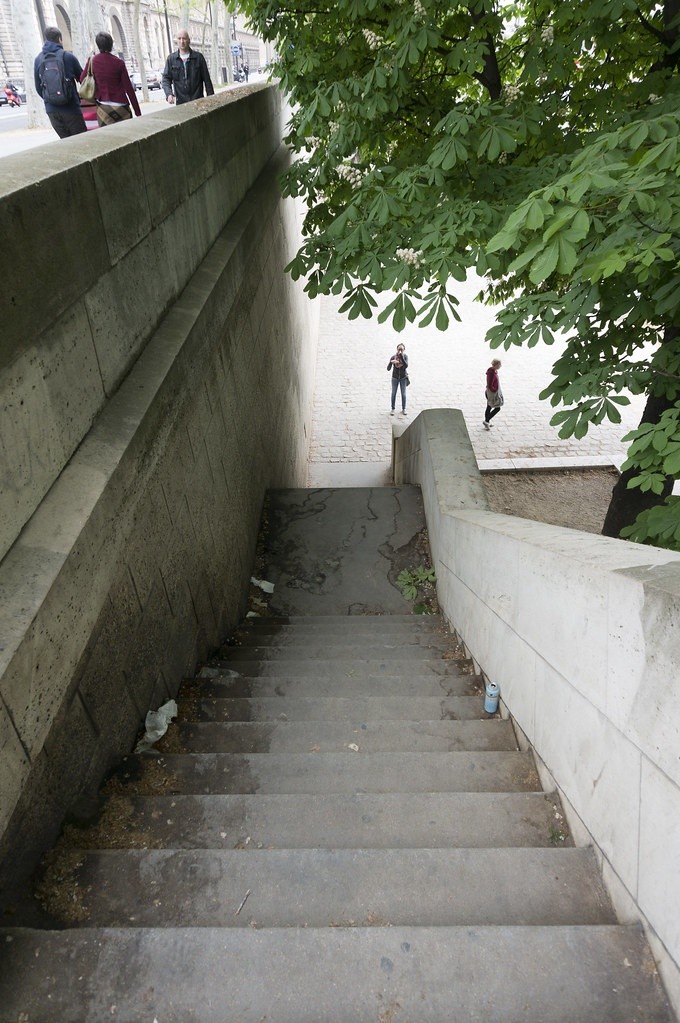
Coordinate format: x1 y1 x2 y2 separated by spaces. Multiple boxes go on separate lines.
233 16 238 72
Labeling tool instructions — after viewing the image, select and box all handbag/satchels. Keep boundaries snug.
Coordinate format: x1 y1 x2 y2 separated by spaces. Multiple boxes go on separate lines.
406 375 410 386
78 55 97 103
487 384 504 407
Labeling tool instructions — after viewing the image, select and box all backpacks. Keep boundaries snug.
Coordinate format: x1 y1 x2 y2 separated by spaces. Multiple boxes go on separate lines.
38 50 75 106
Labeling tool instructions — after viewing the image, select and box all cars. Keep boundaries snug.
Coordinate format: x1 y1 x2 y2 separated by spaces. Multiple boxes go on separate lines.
233 70 245 83
258 66 272 74
130 72 142 89
145 71 163 90
0 84 26 106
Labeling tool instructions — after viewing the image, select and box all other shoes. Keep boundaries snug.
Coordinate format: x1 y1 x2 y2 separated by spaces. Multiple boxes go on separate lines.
391 409 396 414
402 409 407 414
489 423 494 427
483 421 490 430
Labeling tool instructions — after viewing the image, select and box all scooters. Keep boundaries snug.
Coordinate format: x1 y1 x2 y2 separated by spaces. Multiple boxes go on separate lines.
5 88 22 108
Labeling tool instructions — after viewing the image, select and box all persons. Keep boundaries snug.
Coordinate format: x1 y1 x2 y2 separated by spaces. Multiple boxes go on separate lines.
34 26 88 139
387 344 409 415
266 60 269 67
4 80 20 101
130 74 137 90
162 31 214 106
483 359 504 431
258 67 262 74
239 62 249 82
80 31 141 128
